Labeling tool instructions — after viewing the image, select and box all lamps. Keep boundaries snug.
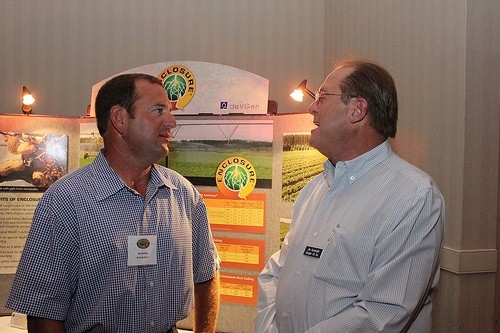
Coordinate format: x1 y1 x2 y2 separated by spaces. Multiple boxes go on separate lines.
21 86 34 114
290 78 316 103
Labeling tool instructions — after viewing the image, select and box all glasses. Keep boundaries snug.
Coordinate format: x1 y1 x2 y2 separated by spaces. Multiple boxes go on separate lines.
315 90 360 105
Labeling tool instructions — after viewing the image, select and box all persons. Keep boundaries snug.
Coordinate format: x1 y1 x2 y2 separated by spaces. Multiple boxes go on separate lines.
255 61 445 333
5 73 222 333
